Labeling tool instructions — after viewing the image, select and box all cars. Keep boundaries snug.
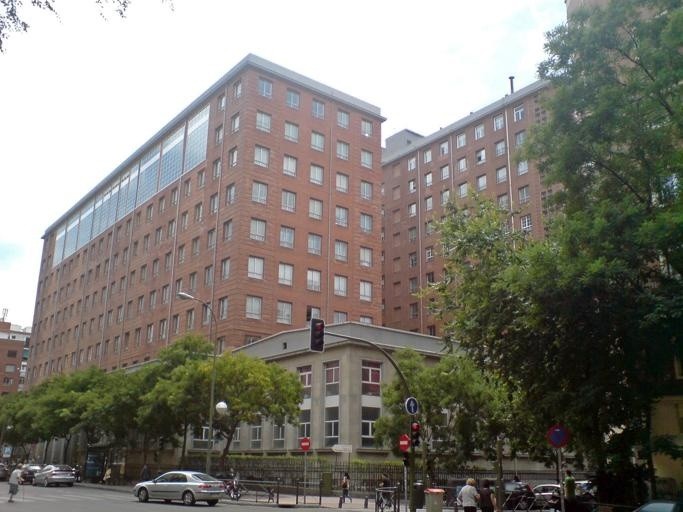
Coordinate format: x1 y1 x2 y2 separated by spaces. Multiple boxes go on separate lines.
490 480 595 509
0 463 75 487
132 471 224 505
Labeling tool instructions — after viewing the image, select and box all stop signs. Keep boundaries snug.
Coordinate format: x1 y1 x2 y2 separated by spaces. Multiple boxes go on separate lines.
398 434 410 451
300 438 311 452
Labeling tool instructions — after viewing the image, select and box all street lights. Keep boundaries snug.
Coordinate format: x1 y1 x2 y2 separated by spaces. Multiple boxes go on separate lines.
175 291 228 474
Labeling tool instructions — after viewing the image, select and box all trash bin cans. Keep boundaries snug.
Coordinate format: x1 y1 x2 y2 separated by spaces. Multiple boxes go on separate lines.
424 488 446 512
414 483 424 509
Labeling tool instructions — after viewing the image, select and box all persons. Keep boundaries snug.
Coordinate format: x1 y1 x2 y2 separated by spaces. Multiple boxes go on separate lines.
476 478 498 512
338 472 352 503
377 472 392 508
454 476 482 511
102 465 111 484
137 463 152 482
70 460 80 481
4 461 25 502
561 467 576 500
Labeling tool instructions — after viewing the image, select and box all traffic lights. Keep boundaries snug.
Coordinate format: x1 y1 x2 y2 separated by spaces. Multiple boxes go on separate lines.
309 317 324 354
408 420 421 446
